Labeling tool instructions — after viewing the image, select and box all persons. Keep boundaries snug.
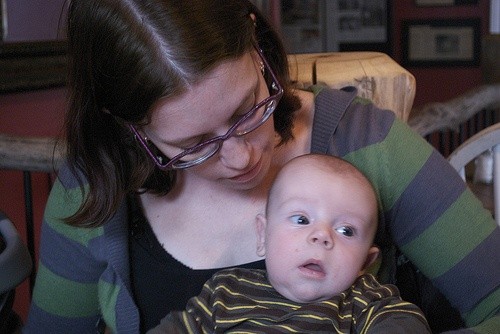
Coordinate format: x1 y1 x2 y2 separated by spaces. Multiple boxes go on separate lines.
22 1 500 334
147 153 434 334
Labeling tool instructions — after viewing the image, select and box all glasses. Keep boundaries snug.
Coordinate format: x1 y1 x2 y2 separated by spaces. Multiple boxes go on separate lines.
129 38 284 170
